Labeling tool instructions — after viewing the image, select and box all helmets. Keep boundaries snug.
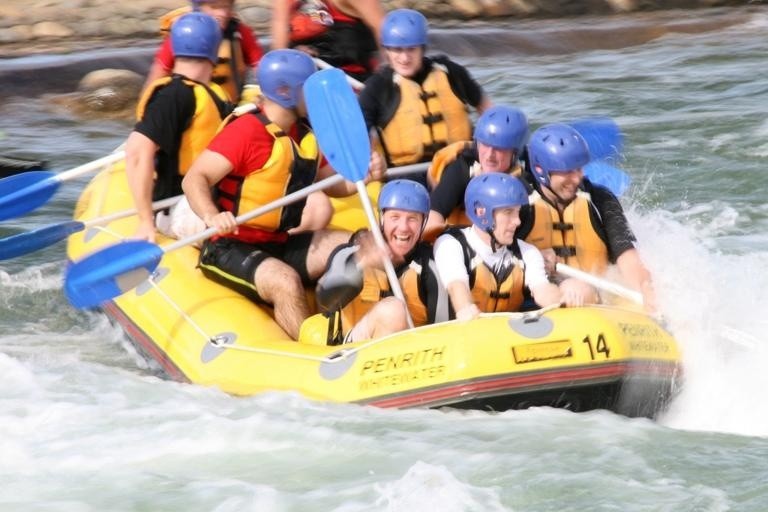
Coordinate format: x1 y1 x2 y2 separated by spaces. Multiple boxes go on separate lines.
256 49 317 107
464 173 530 230
474 106 529 150
170 12 222 60
527 123 591 186
379 179 430 219
380 8 430 48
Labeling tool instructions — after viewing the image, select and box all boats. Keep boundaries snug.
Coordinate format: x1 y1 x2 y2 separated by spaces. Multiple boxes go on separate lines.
67 137 687 418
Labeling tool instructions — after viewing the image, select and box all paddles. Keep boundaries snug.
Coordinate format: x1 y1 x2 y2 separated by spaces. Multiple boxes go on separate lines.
0 149 134 221
312 50 633 205
380 118 621 176
0 194 184 260
303 69 414 330
61 174 341 307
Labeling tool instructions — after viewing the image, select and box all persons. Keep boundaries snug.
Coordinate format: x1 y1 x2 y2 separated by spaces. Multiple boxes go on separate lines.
434 172 565 324
181 48 354 341
124 13 259 244
270 0 385 81
314 179 455 346
520 122 661 325
139 0 265 105
420 106 536 245
360 8 496 182
